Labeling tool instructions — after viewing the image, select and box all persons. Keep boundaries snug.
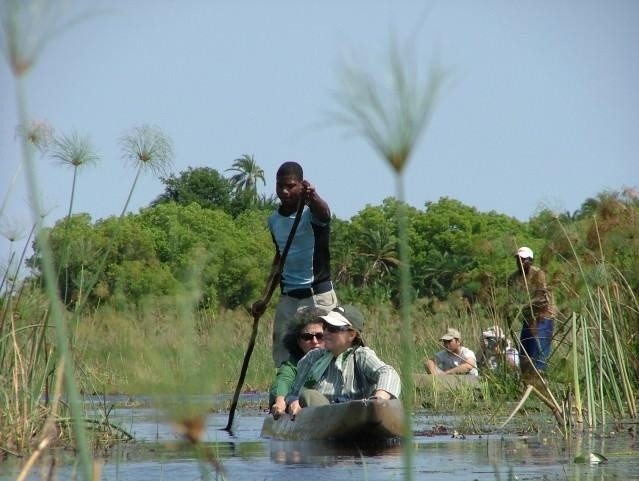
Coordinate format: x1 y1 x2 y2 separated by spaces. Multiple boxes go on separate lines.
424 247 554 374
251 162 401 417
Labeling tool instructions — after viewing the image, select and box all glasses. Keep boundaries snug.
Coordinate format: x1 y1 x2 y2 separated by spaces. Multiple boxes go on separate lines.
322 323 342 333
299 332 324 342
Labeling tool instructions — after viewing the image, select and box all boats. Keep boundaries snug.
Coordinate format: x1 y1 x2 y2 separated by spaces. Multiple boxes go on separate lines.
262 398 405 441
411 373 487 409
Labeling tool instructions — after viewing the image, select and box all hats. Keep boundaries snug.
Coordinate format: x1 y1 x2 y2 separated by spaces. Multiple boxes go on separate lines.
438 328 461 342
478 326 507 350
514 246 534 259
317 303 365 331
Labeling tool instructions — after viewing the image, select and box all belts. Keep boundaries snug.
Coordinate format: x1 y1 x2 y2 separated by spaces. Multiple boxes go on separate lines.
281 278 332 300
